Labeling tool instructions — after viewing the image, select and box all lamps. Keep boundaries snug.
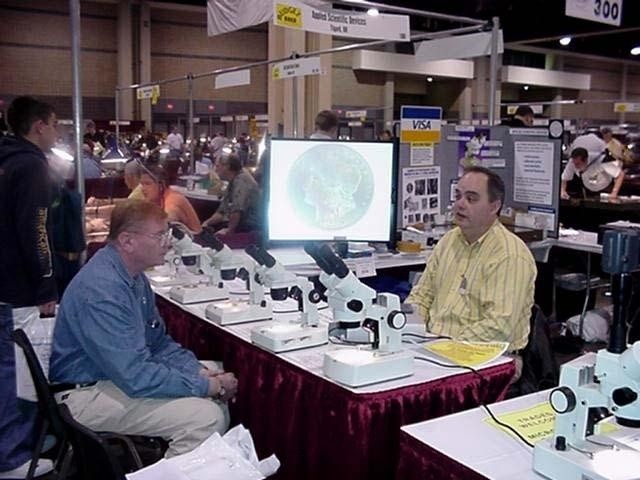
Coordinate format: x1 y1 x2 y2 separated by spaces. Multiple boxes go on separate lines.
51 143 75 163
559 36 570 45
366 7 379 16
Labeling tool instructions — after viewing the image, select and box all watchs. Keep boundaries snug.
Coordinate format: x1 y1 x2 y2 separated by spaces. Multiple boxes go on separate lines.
212 377 227 403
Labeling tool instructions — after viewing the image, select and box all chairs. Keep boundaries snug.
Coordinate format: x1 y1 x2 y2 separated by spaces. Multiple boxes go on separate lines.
11 326 161 478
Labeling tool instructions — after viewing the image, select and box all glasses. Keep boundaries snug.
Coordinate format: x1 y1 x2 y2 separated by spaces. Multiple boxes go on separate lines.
126 226 175 249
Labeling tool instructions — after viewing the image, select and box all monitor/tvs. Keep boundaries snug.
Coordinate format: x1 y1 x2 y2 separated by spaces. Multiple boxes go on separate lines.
265 135 401 253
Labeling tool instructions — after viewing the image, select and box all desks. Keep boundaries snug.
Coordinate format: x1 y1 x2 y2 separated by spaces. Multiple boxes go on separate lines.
64 161 221 263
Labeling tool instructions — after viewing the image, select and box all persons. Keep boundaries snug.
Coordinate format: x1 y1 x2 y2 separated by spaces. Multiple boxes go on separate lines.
571 130 606 154
375 130 392 141
306 110 338 140
399 165 539 386
0 94 62 479
48 197 240 458
497 105 534 127
55 121 264 233
603 128 635 165
560 146 627 204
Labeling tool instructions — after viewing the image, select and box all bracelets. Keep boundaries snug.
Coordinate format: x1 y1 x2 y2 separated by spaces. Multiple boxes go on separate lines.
612 188 618 193
